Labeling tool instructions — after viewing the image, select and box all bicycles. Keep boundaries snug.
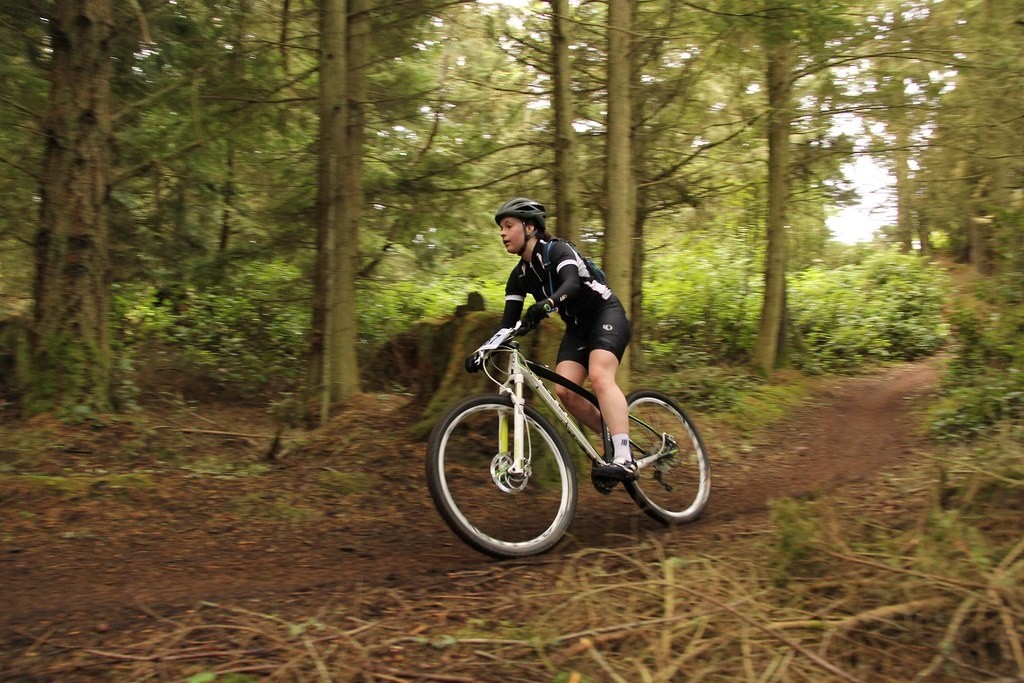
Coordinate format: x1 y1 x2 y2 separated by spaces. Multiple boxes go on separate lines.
425 304 711 559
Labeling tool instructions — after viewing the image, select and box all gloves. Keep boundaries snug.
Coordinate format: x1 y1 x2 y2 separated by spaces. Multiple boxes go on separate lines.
513 299 553 336
465 353 489 373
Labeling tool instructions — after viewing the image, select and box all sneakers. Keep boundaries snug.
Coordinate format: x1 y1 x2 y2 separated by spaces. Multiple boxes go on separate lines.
594 440 614 463
590 459 640 481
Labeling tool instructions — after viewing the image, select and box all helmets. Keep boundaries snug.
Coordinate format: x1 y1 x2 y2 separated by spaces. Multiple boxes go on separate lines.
495 197 547 256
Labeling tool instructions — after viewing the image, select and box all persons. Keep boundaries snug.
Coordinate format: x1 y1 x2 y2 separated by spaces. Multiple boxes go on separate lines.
464 196 641 481
